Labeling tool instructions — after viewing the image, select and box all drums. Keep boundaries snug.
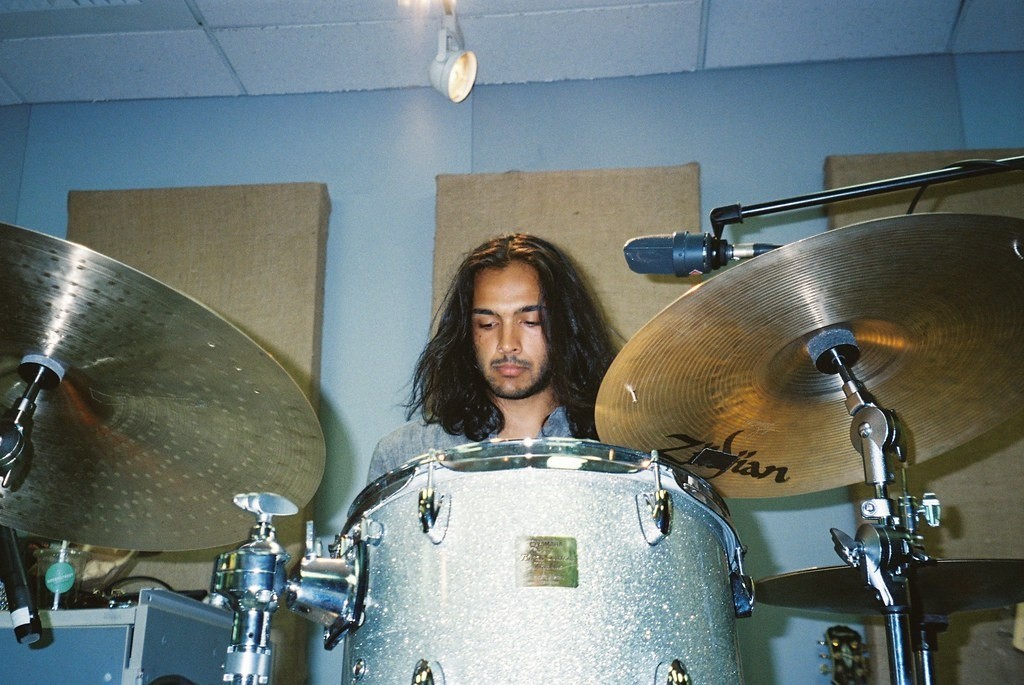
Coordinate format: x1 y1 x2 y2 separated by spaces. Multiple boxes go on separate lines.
341 434 747 684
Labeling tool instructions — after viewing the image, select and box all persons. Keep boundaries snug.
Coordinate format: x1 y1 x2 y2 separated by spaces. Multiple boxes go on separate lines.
366 232 630 487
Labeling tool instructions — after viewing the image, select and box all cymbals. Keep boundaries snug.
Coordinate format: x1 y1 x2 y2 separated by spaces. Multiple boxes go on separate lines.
742 556 1024 618
1 226 331 556
593 209 1011 502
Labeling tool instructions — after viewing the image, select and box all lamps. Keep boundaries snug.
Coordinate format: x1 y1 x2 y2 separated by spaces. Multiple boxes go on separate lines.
430 26 479 104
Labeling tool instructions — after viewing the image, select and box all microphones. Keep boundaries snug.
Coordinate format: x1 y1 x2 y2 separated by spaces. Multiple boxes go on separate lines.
624 231 782 277
